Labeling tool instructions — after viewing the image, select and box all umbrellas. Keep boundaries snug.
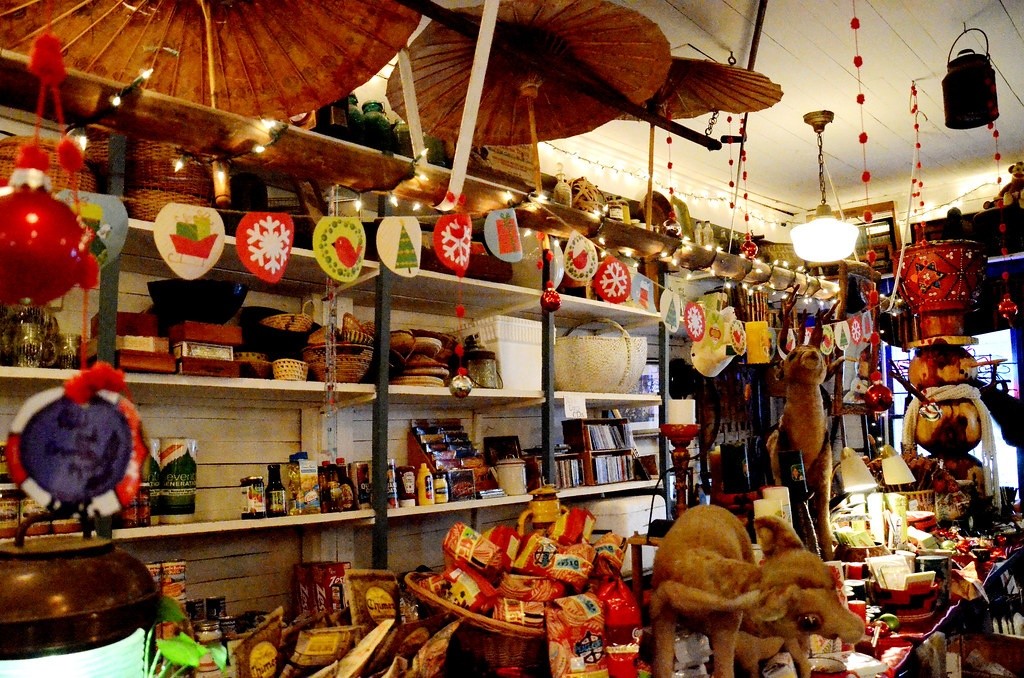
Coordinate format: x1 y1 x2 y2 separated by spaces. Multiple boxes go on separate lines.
0 0 421 205
388 0 670 251
616 57 784 231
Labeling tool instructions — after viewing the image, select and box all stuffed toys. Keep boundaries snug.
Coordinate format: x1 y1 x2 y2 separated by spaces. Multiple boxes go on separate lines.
998 162 1024 208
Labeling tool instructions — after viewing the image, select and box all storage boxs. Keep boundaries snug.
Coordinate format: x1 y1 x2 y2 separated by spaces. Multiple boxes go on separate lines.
570 494 668 575
449 314 558 394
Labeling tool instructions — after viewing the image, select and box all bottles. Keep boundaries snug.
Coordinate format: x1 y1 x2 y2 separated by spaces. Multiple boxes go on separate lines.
694 221 739 255
605 195 630 225
318 458 354 512
395 463 448 506
266 451 309 518
320 93 448 166
553 162 572 208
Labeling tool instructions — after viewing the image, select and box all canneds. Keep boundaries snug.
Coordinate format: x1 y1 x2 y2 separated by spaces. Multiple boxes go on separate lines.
0 437 199 538
239 476 266 520
433 474 448 504
186 595 237 672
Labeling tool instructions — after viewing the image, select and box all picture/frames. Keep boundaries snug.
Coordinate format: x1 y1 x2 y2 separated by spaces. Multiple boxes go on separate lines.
603 358 672 439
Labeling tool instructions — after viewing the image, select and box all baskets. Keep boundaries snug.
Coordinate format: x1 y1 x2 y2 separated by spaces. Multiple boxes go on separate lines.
404 572 543 670
556 314 648 393
263 301 458 381
0 121 211 223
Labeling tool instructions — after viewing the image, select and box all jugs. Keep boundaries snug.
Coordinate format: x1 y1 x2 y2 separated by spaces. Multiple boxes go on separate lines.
490 453 528 495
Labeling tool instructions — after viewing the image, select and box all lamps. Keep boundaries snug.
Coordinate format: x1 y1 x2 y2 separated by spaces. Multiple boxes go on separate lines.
790 111 862 262
828 448 877 493
870 445 915 486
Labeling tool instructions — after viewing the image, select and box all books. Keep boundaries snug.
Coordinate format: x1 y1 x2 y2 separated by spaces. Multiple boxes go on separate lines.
416 424 505 501
585 424 633 450
593 454 634 484
537 458 585 488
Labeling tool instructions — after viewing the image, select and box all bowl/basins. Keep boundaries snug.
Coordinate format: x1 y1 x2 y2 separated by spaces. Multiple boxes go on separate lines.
635 191 673 228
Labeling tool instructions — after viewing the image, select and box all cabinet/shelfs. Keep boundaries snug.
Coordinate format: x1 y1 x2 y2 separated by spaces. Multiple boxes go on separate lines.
0 51 675 582
